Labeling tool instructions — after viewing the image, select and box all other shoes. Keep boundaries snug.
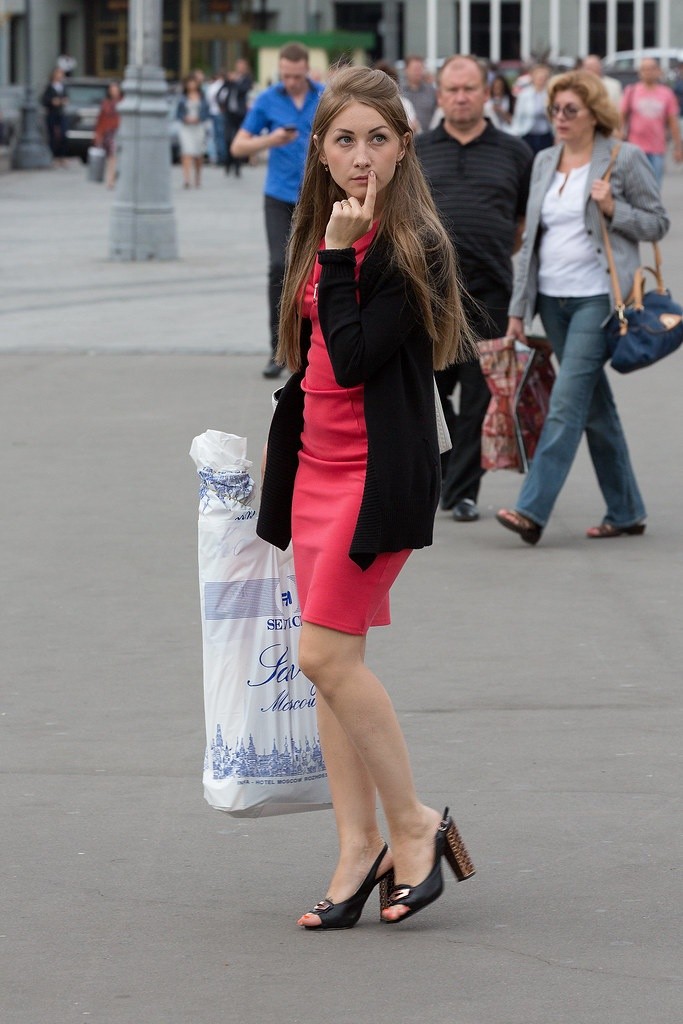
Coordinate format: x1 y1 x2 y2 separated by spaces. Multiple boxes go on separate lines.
262 357 286 378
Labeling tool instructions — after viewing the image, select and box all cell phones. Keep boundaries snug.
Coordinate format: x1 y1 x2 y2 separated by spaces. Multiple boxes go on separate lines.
284 124 297 133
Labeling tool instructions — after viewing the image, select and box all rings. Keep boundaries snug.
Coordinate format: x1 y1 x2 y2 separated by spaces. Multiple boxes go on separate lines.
341 201 352 208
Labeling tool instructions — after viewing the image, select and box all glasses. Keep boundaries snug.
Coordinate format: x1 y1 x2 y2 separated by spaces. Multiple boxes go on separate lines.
547 103 587 120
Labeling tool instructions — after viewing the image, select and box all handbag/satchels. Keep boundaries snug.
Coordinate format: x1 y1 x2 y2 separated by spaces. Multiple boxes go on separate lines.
475 334 556 473
610 288 683 374
189 427 332 817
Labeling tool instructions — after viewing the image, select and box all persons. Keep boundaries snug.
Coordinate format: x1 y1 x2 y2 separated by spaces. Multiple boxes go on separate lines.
39 67 70 163
93 81 122 189
496 71 671 546
171 58 254 187
231 44 326 377
376 54 683 187
257 65 478 929
412 54 534 521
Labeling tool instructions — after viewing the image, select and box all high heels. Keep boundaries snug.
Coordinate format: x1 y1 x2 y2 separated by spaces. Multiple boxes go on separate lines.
306 842 394 930
381 806 475 923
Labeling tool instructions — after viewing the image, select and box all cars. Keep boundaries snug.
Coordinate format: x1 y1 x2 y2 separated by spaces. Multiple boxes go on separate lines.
60 76 120 141
605 50 682 92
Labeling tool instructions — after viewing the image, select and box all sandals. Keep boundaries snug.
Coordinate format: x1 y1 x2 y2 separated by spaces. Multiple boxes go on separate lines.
494 509 541 545
587 524 647 538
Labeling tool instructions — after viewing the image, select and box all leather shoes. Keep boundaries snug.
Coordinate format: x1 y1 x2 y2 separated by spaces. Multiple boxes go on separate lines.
454 502 478 521
